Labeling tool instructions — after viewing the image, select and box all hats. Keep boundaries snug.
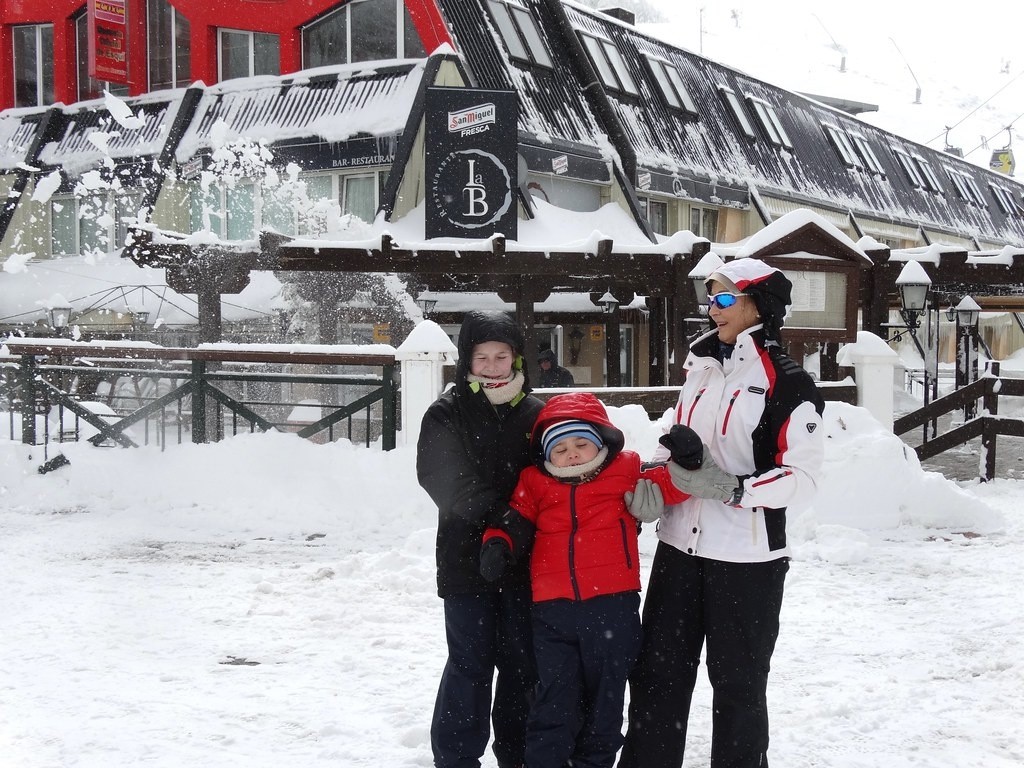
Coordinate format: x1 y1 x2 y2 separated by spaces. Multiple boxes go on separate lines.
541 419 603 462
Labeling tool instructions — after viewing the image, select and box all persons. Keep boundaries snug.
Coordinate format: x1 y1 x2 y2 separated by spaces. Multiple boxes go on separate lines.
537 349 576 388
617 257 827 768
480 388 707 768
414 307 550 768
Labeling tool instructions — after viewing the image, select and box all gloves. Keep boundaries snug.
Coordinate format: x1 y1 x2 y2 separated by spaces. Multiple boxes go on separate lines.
668 443 739 502
480 542 517 583
481 498 537 550
624 479 665 523
659 424 703 471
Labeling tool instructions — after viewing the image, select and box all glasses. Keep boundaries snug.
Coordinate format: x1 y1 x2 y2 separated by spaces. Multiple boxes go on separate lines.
539 360 548 364
707 292 750 311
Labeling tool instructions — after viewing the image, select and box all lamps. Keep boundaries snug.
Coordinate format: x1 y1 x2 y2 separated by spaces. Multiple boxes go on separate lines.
597 292 619 322
43 293 73 330
880 259 932 343
568 326 584 365
945 294 981 336
135 306 150 324
417 286 439 321
